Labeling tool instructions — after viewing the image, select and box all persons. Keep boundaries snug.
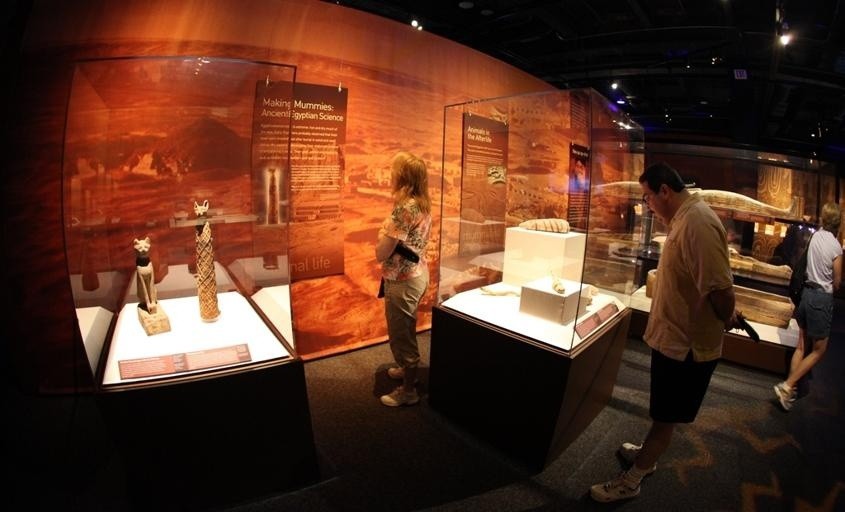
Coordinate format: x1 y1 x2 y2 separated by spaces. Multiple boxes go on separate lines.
588 162 738 501
772 222 814 273
374 151 432 407
771 201 843 413
569 157 590 193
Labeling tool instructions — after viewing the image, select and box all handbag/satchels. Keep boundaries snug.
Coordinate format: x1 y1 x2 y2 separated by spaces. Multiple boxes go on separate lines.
787 230 818 308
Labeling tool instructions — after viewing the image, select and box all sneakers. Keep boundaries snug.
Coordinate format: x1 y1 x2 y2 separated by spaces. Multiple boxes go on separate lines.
621 441 658 475
380 385 419 407
773 380 797 412
387 367 418 383
588 472 642 504
